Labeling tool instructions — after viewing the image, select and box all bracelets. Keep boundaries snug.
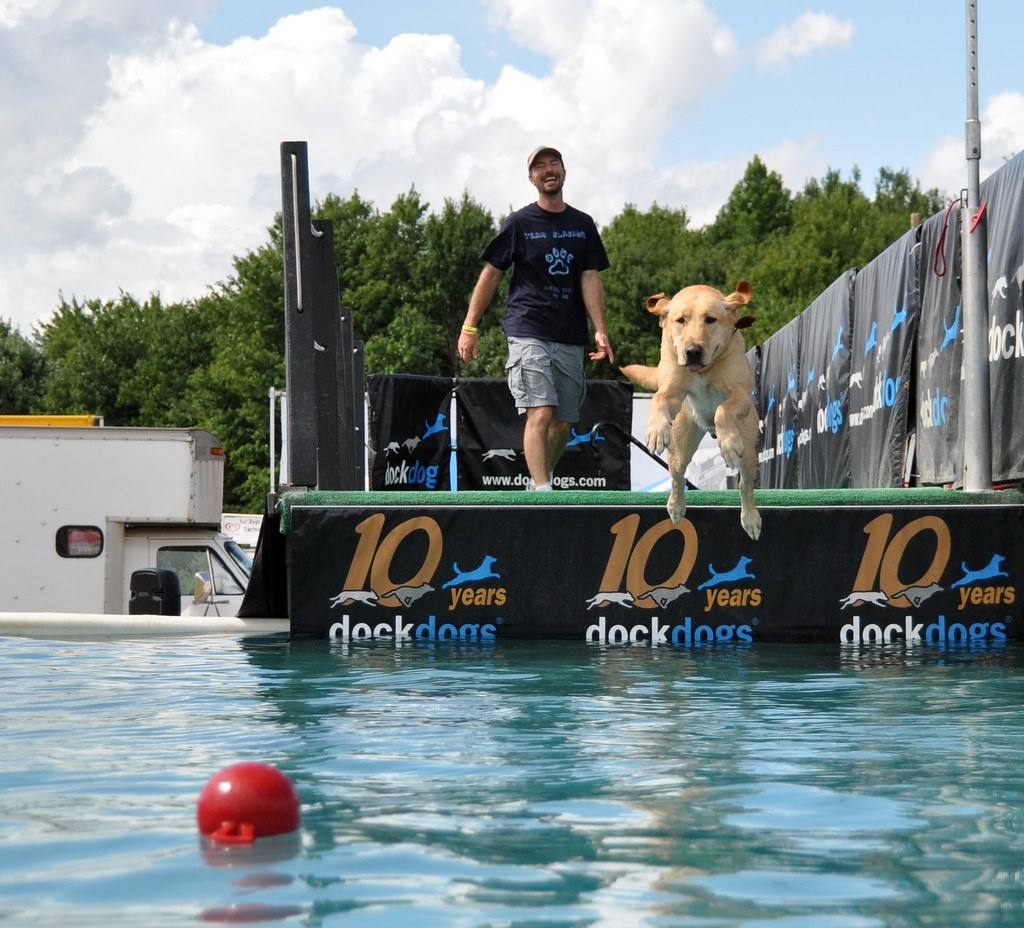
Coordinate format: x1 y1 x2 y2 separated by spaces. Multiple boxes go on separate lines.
462 325 478 332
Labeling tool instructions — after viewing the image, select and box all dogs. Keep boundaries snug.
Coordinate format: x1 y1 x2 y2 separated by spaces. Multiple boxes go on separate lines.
617 278 762 540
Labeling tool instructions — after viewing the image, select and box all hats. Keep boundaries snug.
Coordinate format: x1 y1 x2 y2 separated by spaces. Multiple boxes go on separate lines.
527 144 563 171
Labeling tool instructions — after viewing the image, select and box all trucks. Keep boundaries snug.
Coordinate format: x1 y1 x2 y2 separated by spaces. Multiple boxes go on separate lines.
1 420 254 617
223 514 264 560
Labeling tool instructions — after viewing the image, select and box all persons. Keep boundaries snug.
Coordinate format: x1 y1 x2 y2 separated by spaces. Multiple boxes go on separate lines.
457 146 615 490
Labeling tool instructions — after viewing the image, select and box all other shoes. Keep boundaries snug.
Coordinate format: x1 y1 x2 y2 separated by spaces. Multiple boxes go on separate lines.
525 475 555 490
